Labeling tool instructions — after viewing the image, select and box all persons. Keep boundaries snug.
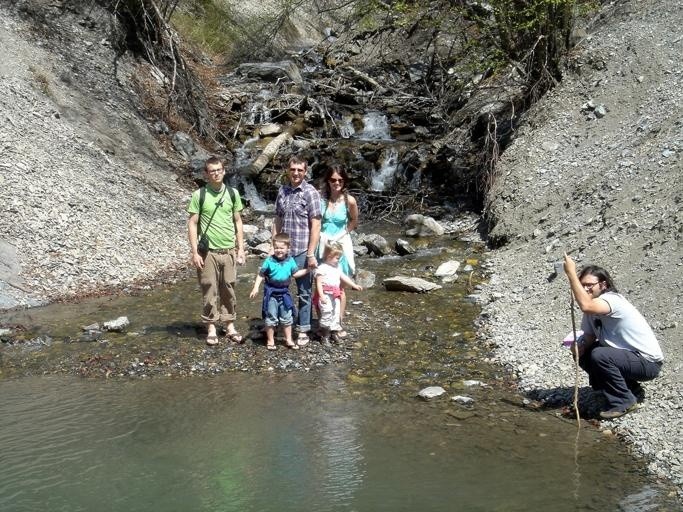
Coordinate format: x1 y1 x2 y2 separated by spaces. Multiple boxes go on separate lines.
273 156 323 348
312 165 359 339
248 232 311 351
561 250 665 419
187 156 245 346
313 239 365 346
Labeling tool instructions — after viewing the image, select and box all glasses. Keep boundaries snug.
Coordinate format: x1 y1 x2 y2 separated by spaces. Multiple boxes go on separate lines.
582 282 599 289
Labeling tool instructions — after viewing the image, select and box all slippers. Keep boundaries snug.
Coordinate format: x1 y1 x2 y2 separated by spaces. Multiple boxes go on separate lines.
206 335 219 346
266 333 347 351
230 334 244 343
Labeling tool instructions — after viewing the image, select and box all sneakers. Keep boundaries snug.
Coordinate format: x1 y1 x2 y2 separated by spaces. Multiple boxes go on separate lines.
600 402 637 419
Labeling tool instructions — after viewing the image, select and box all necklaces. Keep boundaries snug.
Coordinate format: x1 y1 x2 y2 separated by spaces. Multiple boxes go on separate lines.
334 199 337 207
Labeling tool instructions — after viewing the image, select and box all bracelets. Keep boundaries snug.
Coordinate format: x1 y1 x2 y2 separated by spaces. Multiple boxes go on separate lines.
306 253 316 259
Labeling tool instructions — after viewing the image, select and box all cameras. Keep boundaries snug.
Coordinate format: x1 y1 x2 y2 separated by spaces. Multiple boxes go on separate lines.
198 238 209 252
